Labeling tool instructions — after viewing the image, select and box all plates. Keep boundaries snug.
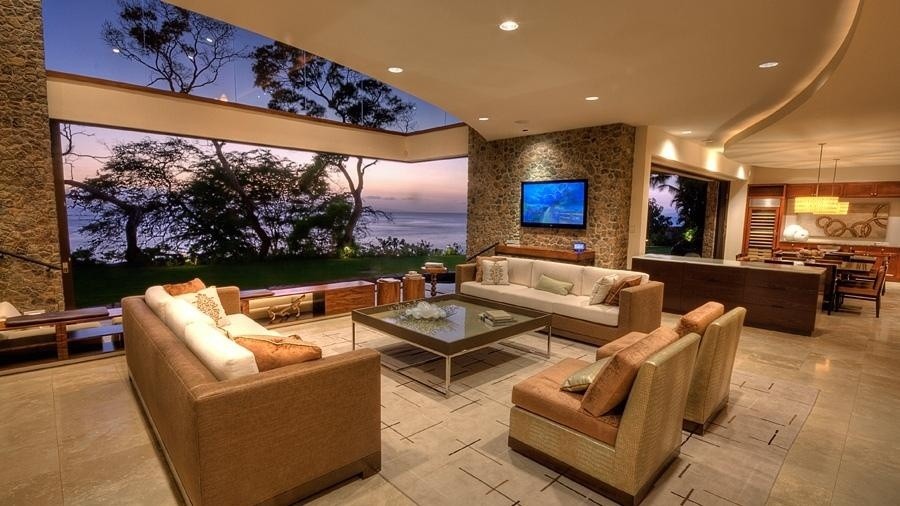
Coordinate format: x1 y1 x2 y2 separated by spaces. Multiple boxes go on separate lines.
832 252 854 255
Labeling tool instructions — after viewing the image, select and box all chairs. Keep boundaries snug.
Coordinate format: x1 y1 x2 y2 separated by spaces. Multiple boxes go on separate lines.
596 306 747 436
508 332 701 505
735 243 891 318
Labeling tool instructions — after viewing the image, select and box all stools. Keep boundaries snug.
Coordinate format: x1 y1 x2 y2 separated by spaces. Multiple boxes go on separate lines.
402 277 426 302
376 279 400 306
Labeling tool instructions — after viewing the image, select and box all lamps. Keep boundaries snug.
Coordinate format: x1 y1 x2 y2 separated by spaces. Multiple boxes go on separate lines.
794 142 850 215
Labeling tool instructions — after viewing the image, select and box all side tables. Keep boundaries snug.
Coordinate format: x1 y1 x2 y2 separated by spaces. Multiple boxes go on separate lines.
421 265 449 296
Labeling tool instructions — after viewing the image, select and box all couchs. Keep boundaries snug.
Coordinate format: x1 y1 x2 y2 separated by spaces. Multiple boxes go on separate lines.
455 258 664 348
120 285 382 506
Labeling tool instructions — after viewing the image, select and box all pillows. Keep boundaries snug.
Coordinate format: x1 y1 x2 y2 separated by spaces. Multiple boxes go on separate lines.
588 275 618 305
604 275 642 306
559 357 613 392
581 327 680 418
475 255 506 282
534 272 574 297
144 278 322 383
675 301 725 339
481 259 510 285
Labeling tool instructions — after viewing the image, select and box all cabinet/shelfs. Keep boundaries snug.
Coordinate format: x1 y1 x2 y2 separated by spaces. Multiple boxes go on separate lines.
741 184 900 283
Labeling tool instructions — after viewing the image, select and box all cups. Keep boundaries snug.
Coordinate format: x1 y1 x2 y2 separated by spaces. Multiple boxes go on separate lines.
776 248 783 260
849 246 855 252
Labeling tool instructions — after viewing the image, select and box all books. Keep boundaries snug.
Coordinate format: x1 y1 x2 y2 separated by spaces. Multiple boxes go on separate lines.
477 310 517 325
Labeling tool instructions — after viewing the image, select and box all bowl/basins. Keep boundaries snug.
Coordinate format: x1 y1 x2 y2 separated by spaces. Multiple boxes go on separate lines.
406 274 421 280
818 245 841 251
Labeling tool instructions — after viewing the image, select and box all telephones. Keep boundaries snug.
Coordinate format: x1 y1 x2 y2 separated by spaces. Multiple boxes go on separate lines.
573 243 585 250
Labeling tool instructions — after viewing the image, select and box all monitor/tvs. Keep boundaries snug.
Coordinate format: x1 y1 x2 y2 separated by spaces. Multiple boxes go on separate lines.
521 178 588 228
